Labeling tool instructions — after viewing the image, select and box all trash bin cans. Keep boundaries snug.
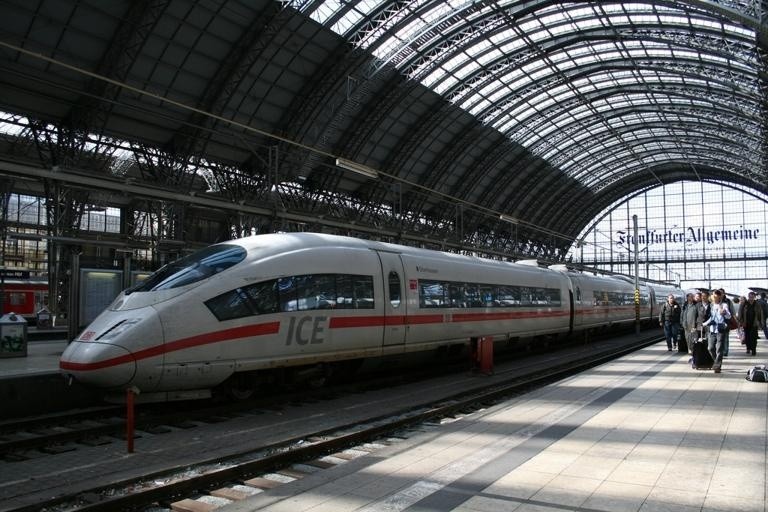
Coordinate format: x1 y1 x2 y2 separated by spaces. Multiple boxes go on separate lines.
36 308 53 330
0 312 28 358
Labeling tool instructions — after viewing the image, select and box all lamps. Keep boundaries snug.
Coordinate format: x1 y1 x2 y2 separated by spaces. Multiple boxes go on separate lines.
335 157 378 179
499 215 519 225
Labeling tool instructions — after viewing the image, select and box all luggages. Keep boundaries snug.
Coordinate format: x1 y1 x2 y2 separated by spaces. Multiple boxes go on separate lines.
691 324 714 370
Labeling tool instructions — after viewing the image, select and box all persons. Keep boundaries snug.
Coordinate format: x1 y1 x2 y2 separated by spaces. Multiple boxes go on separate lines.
658 287 768 373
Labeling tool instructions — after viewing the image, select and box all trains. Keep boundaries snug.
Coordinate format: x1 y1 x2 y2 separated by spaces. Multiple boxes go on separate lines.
0 276 51 318
53 229 742 404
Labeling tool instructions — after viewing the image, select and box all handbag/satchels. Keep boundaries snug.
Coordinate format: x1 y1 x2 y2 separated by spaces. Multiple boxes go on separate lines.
746 367 768 382
724 314 738 330
